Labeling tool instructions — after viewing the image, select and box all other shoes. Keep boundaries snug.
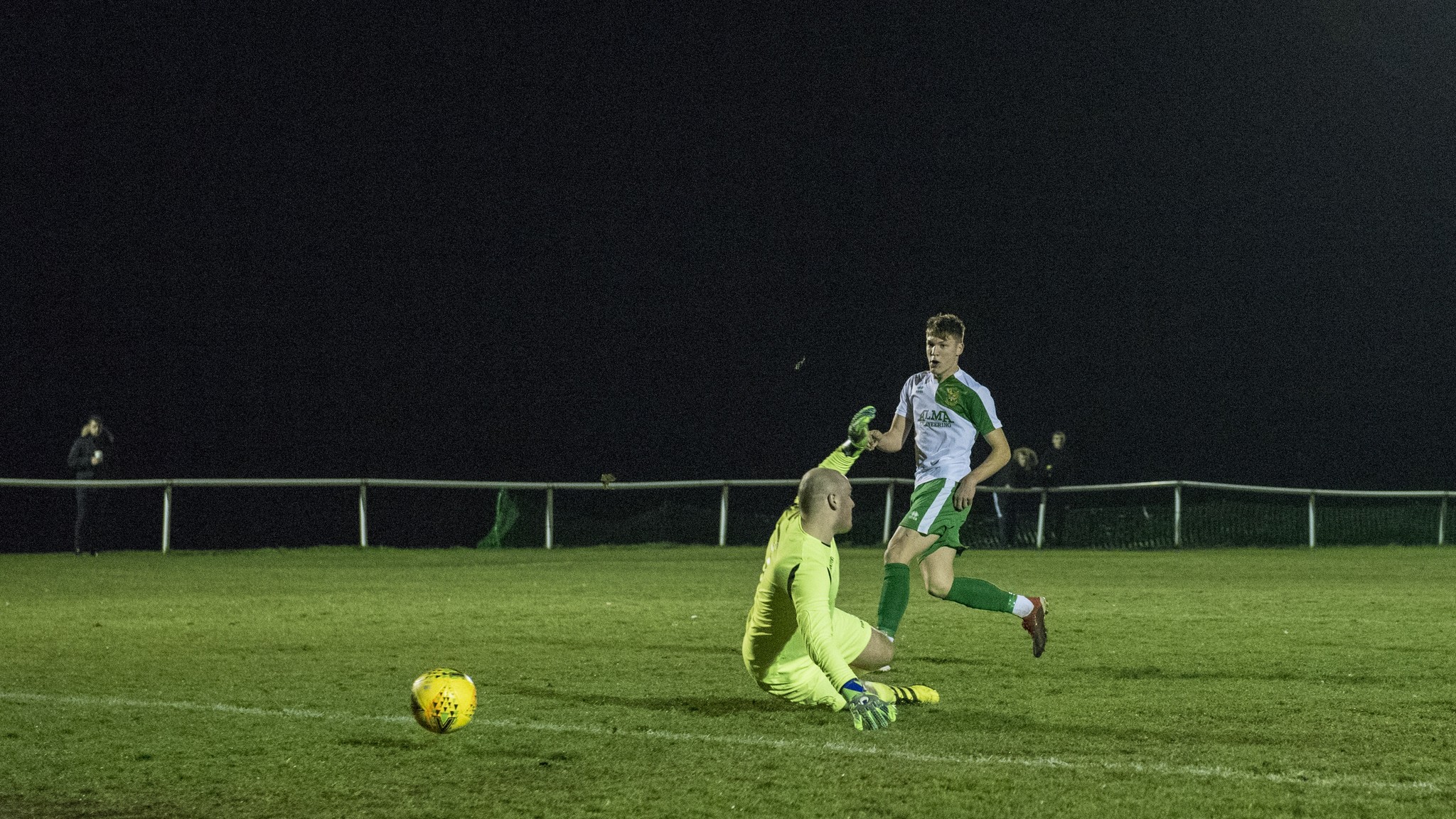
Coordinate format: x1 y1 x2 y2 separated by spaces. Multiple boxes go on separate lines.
74 548 83 556
89 548 98 558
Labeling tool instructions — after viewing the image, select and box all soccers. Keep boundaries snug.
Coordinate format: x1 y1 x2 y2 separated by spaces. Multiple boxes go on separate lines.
409 666 479 735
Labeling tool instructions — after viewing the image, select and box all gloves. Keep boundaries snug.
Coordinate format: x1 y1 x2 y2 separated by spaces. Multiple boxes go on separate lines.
836 405 877 458
839 678 897 731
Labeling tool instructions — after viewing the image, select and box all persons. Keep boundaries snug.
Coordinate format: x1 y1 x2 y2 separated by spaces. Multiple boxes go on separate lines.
991 431 1070 550
743 405 938 731
867 315 1049 674
68 415 120 555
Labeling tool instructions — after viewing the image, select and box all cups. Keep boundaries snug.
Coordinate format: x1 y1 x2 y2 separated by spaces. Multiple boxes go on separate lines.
94 450 103 462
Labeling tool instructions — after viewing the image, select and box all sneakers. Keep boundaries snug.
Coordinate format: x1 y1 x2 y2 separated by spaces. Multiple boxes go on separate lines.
1021 597 1049 658
875 665 891 672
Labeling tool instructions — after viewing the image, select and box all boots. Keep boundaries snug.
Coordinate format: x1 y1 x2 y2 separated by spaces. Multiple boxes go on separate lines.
864 681 939 704
